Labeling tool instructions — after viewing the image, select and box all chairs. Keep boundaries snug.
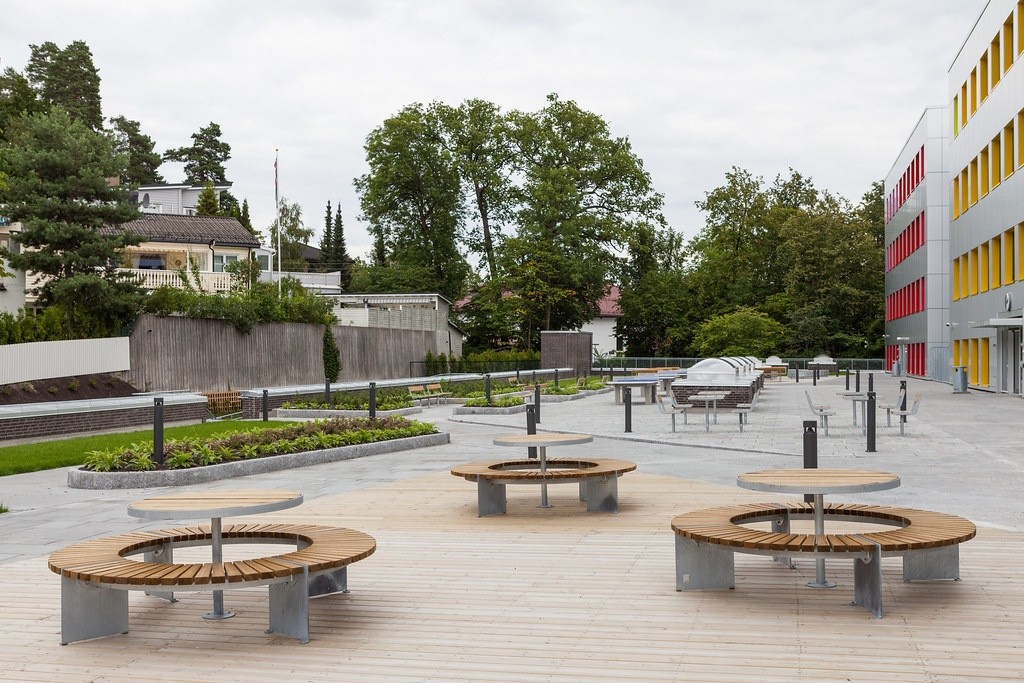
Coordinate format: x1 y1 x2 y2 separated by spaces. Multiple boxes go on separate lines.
655 394 683 432
805 390 836 436
670 390 693 424
891 392 924 435
879 389 906 426
731 392 758 432
736 389 761 423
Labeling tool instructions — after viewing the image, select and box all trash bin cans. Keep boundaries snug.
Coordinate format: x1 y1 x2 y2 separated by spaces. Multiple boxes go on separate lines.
892 361 899 376
951 365 970 394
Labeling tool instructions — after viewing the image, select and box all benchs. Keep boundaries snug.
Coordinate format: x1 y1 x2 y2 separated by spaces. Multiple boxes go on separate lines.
427 384 453 406
48 525 376 645
672 502 978 619
407 385 438 408
508 377 527 386
451 457 638 518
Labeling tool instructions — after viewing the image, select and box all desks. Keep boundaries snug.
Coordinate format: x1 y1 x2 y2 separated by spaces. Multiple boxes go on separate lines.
736 468 901 589
843 396 869 436
607 372 687 406
697 391 731 424
492 433 593 509
688 395 725 432
836 392 865 428
125 489 306 620
